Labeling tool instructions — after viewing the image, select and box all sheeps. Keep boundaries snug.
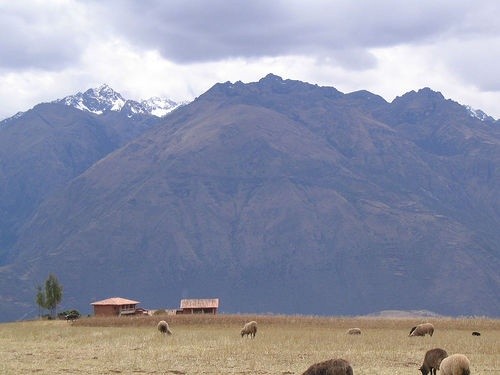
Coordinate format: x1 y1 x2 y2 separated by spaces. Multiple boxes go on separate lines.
158 320 173 334
472 332 480 336
408 326 416 336
303 359 353 375
346 328 362 335
419 349 448 375
410 323 434 337
241 321 258 338
438 354 471 375
